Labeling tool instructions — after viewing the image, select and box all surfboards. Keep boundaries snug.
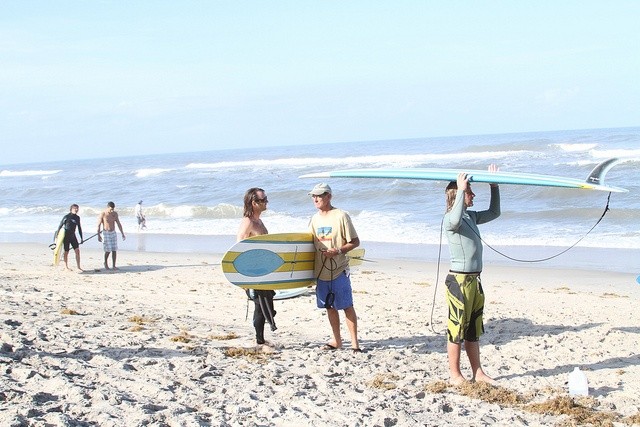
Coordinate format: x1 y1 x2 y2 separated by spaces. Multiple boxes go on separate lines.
298 156 630 194
49 225 65 267
222 232 365 290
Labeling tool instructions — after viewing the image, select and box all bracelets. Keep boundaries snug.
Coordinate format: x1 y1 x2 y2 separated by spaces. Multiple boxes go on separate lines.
336 247 342 255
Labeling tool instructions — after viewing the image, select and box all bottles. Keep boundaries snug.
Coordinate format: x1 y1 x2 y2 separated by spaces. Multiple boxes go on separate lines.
568 365 590 398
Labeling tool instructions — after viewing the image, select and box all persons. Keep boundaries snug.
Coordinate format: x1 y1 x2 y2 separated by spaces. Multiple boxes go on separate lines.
307 183 364 354
443 165 502 387
238 188 277 347
97 201 126 270
56 204 85 273
135 200 148 231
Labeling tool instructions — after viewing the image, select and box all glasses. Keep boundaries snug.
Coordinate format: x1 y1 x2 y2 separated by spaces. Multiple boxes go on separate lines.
255 196 267 203
308 184 331 195
